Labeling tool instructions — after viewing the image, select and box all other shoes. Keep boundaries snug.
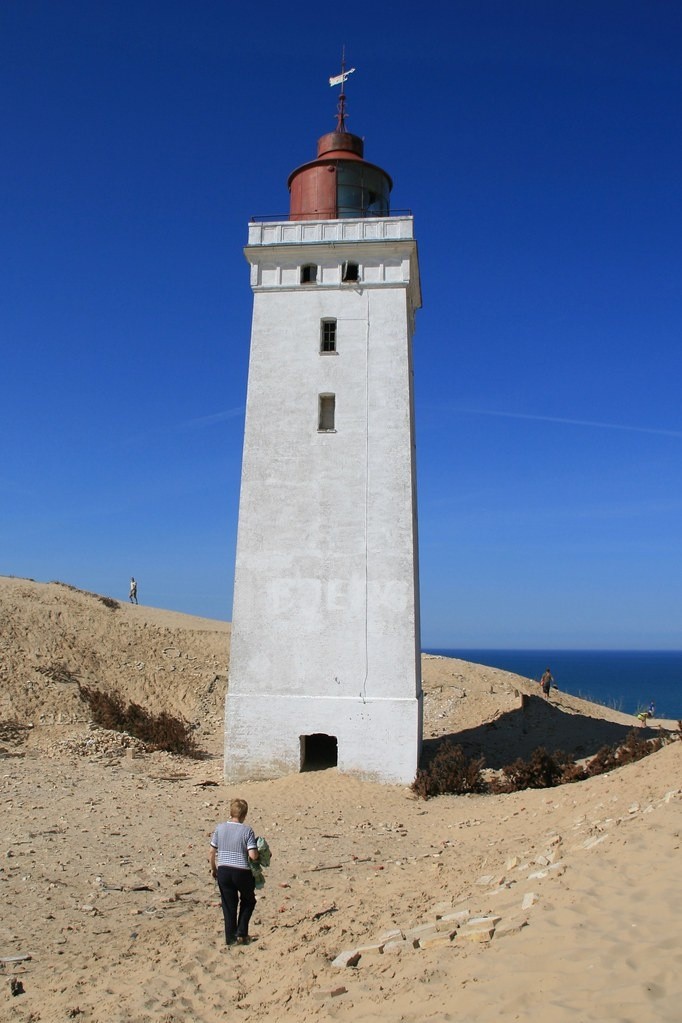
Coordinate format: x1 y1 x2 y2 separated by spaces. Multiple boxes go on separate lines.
237 935 250 945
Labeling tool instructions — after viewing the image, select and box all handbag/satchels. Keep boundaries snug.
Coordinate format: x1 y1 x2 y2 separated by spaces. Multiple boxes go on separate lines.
540 681 544 686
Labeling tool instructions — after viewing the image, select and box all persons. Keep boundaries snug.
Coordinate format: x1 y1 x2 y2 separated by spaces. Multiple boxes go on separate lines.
209 798 260 946
129 577 138 605
541 668 555 701
638 700 654 728
